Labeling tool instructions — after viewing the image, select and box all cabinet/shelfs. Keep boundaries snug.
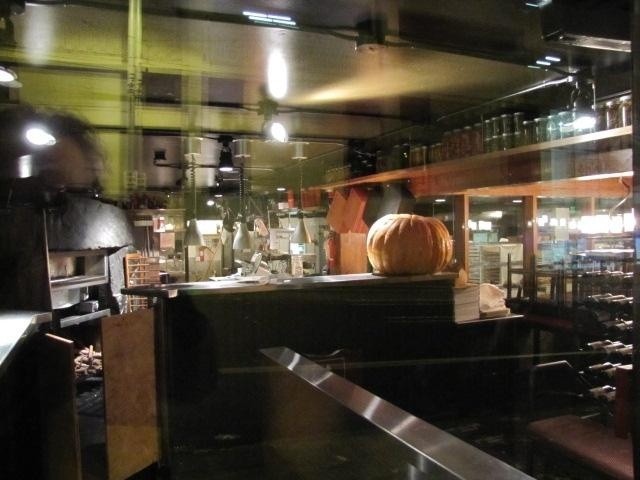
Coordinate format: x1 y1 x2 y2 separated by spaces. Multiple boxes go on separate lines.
1 310 84 480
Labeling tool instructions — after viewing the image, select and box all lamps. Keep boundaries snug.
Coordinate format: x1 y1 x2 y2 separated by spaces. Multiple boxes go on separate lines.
289 141 313 244
181 135 206 246
570 66 597 130
233 139 253 251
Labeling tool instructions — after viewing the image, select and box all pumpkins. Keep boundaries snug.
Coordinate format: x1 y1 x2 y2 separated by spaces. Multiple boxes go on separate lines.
366 212 454 276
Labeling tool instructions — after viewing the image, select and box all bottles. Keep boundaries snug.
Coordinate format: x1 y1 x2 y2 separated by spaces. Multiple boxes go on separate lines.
183 210 312 243
389 95 633 171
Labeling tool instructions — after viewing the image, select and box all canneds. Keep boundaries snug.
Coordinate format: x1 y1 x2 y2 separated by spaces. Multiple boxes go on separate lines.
410 95 634 167
